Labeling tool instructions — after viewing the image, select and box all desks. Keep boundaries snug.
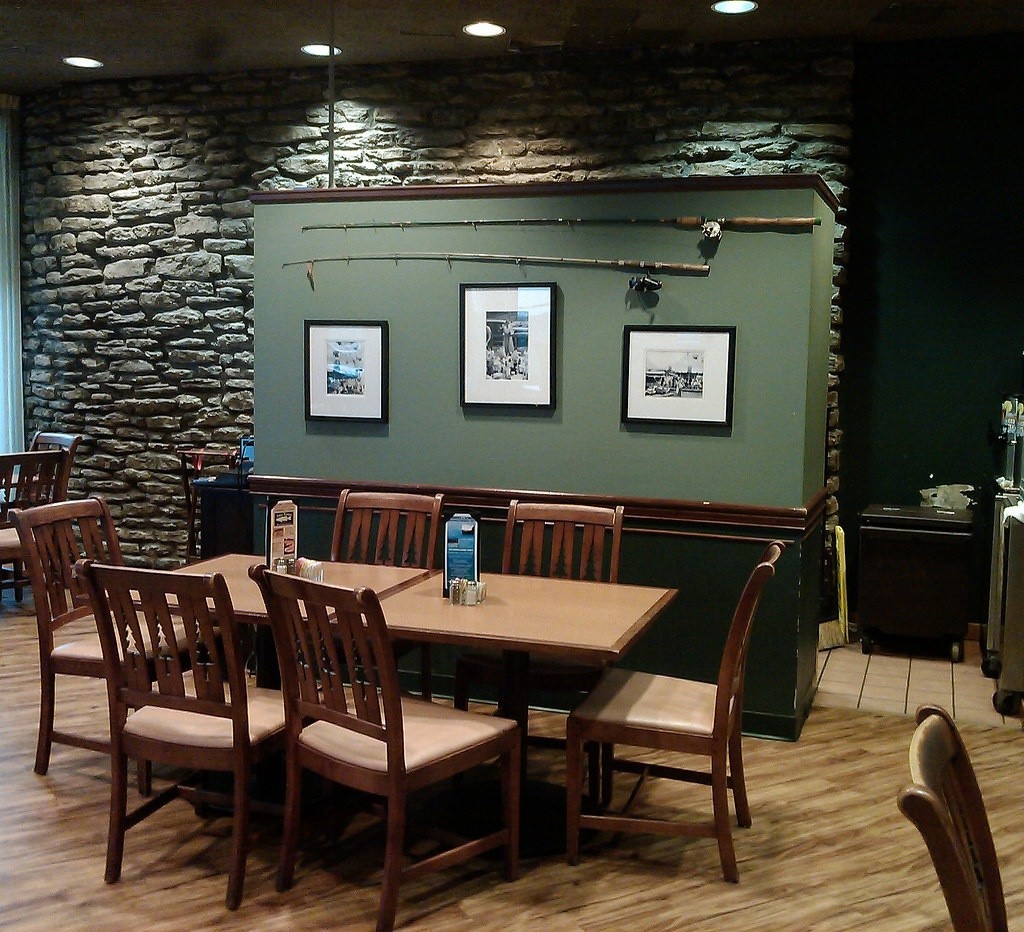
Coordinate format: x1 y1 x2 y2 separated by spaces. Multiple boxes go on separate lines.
105 553 432 628
2 474 39 490
332 569 680 804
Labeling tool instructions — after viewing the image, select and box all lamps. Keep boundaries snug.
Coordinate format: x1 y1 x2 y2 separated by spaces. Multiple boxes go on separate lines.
628 276 661 292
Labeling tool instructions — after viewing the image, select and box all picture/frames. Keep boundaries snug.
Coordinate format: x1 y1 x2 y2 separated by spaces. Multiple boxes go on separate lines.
303 319 390 424
622 323 736 431
458 280 557 411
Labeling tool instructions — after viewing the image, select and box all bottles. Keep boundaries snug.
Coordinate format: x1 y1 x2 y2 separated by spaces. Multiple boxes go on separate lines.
464 581 478 606
276 559 287 574
450 580 462 605
287 559 295 575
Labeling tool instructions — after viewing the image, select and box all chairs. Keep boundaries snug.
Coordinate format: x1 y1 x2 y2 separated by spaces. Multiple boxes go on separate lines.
329 489 445 570
13 430 84 601
6 493 200 799
245 559 520 932
0 449 71 611
895 701 1011 932
72 558 310 912
561 537 787 882
500 499 627 801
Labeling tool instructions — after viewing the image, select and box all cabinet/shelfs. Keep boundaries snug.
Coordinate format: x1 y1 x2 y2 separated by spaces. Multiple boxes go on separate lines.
199 486 253 560
854 501 976 663
